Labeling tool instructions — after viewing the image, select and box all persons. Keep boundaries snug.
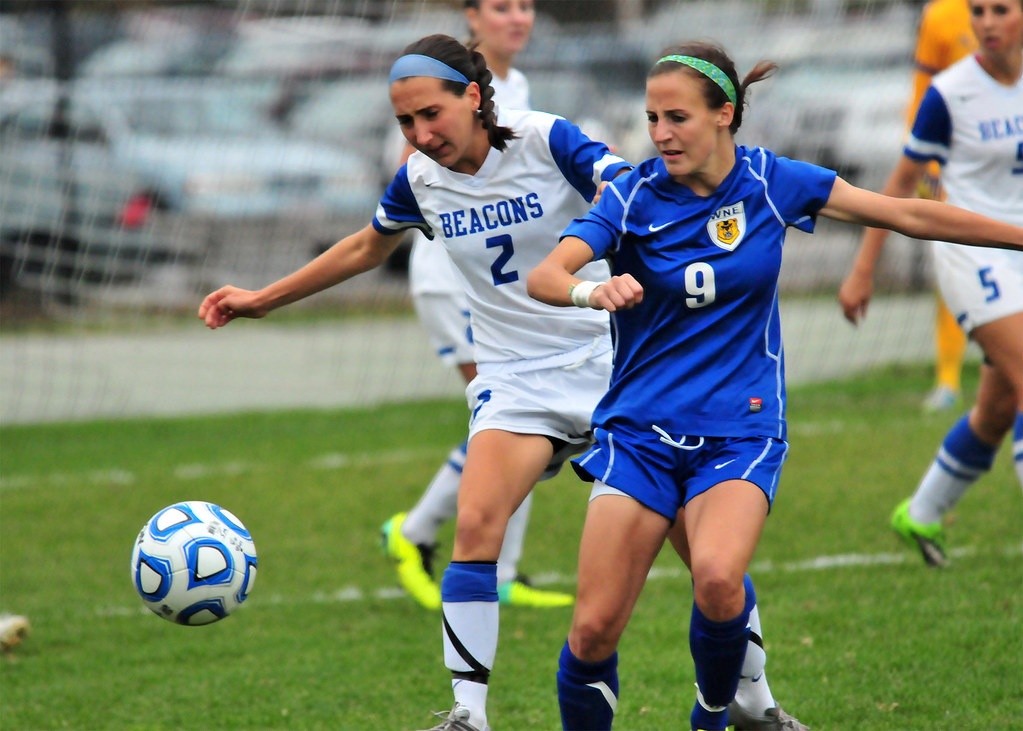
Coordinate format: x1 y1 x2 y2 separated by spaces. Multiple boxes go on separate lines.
838 0 1023 571
526 42 1023 731
196 0 693 731
0 614 31 652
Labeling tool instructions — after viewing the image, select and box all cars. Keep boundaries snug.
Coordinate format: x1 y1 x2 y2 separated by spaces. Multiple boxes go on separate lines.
1 2 916 294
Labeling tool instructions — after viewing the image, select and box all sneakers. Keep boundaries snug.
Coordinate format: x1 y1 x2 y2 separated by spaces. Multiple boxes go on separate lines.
497 574 574 608
426 702 479 731
381 511 443 610
725 697 810 731
889 499 953 569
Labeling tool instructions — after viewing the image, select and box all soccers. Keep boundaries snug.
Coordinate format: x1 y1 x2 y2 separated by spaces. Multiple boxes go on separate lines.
128 499 260 626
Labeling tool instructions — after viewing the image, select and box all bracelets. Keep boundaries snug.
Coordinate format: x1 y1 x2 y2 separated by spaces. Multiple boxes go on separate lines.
571 281 605 310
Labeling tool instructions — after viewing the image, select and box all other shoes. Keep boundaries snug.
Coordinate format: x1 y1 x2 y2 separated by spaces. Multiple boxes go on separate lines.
924 392 956 413
